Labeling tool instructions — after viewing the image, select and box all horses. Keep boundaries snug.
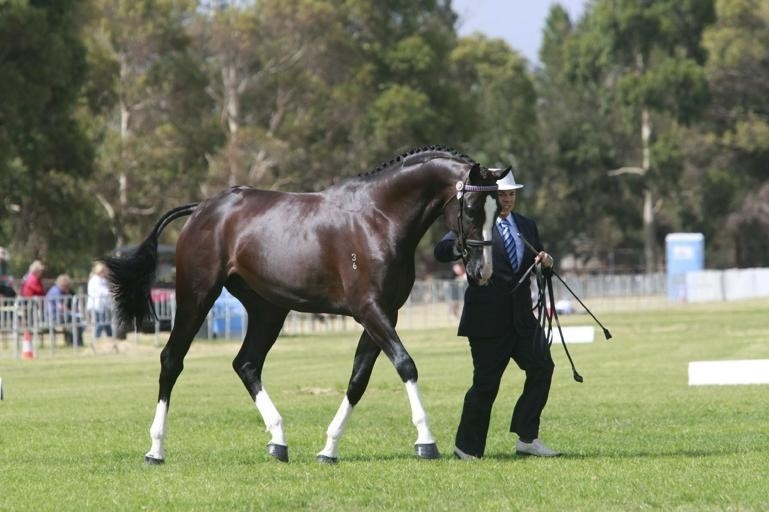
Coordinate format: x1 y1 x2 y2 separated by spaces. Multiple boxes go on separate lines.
93 144 512 465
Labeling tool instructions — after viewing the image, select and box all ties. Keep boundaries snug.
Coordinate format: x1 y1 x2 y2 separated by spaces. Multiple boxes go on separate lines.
498 217 517 277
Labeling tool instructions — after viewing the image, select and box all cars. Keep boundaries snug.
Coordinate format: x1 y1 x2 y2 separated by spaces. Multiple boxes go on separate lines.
112 244 177 332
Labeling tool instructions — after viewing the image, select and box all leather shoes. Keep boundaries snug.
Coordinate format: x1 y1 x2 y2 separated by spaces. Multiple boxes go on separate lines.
517 440 564 457
453 446 479 460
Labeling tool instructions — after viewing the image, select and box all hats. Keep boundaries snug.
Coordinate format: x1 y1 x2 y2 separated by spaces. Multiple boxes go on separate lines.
487 168 524 191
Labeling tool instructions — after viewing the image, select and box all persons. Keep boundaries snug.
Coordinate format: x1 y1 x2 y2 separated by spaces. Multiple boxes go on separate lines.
0 250 116 348
434 183 561 461
450 258 471 318
